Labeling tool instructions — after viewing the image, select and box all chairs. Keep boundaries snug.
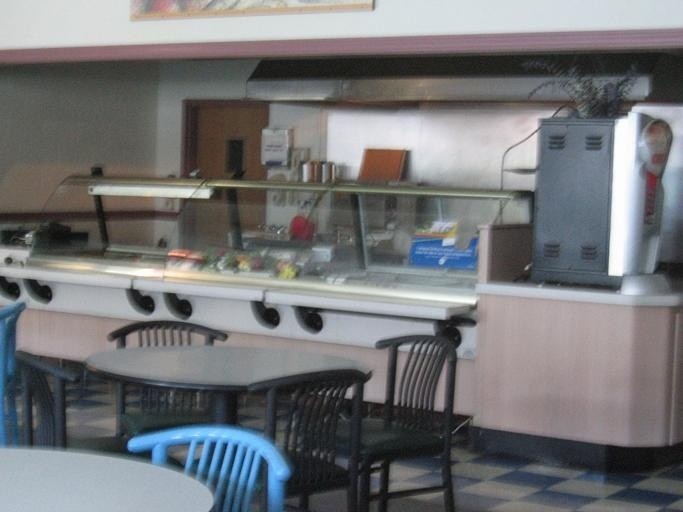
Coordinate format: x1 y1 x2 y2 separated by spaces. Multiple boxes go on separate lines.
1 301 27 446
127 425 291 512
14 349 79 453
106 319 230 436
247 368 373 512
300 333 460 511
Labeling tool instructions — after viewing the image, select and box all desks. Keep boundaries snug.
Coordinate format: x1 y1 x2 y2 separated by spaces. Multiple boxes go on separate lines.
1 446 215 512
86 345 373 427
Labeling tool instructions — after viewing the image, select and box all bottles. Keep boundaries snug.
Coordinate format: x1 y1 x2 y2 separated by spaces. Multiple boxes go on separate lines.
298 161 336 185
